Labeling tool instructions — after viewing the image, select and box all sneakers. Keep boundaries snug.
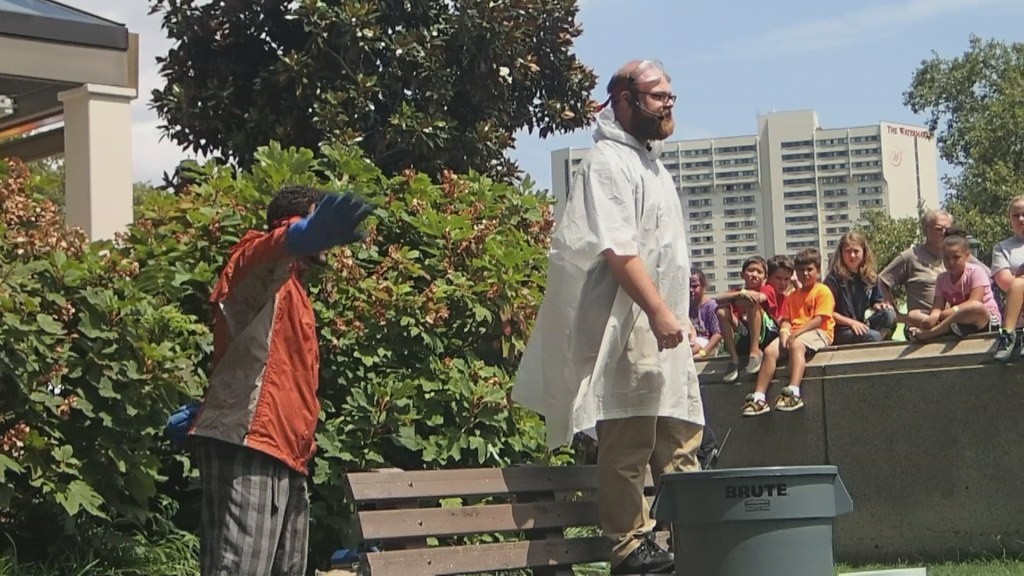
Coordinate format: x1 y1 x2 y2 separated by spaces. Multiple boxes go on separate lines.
611 538 675 573
994 327 1024 359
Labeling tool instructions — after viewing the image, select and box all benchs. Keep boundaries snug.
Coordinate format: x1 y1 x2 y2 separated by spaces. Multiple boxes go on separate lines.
345 463 673 576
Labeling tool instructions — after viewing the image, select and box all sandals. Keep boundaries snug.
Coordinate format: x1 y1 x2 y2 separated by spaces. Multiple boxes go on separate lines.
774 386 803 411
950 323 972 337
904 325 922 342
740 399 770 416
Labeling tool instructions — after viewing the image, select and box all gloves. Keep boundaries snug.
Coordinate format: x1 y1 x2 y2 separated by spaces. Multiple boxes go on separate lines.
289 191 373 259
165 402 201 440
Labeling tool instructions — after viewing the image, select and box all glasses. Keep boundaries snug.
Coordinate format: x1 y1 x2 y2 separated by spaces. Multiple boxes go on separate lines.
636 91 676 104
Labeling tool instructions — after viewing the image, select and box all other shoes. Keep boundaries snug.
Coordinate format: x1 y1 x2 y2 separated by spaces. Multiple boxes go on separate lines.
745 349 764 374
722 358 742 382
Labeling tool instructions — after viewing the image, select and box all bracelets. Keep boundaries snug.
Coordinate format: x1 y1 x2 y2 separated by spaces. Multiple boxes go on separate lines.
698 350 706 356
952 305 959 312
740 288 746 299
779 327 790 336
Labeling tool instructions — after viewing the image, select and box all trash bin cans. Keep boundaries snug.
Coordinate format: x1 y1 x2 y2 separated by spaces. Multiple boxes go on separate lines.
650 464 854 576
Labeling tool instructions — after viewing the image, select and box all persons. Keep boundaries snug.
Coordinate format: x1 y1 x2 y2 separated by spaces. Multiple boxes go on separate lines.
511 60 706 572
714 256 778 382
742 248 835 416
990 196 1024 361
187 185 371 576
907 234 1002 341
823 232 896 345
764 254 802 326
689 268 724 377
878 209 994 341
